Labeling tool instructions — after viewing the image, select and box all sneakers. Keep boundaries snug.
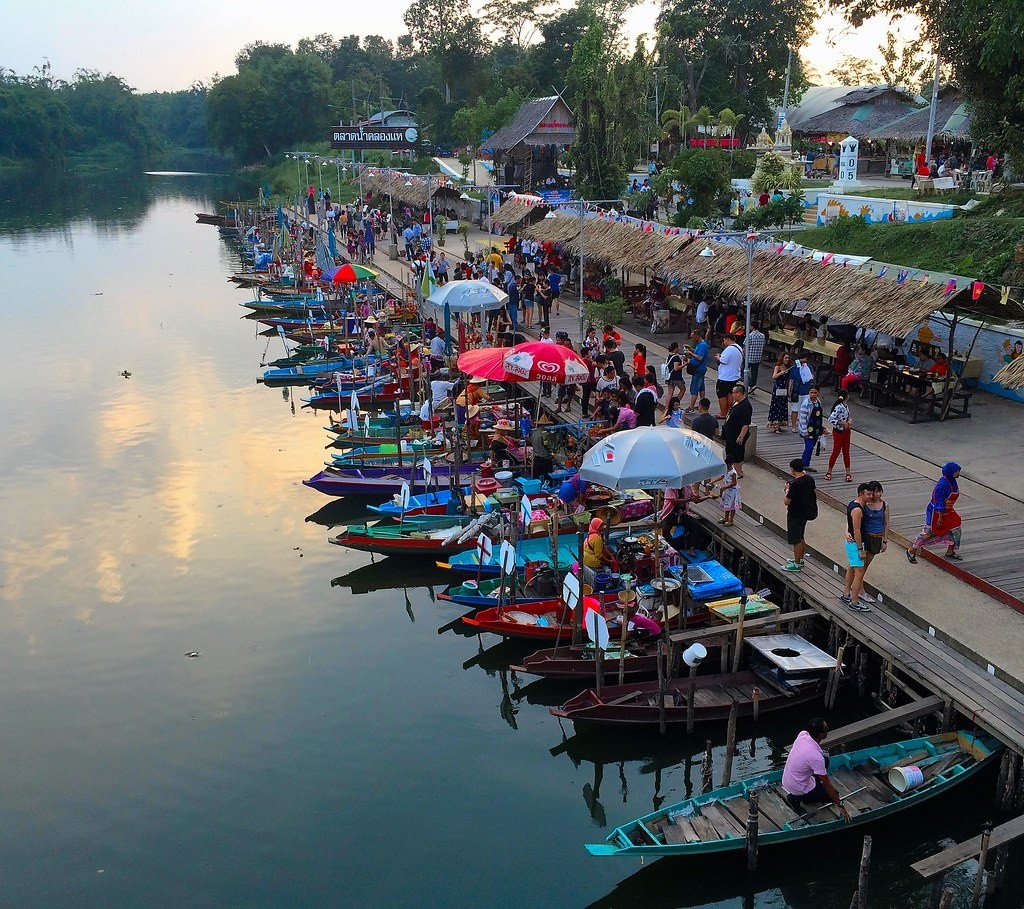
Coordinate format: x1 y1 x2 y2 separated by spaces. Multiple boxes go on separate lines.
780 558 804 572
841 594 870 612
683 406 698 414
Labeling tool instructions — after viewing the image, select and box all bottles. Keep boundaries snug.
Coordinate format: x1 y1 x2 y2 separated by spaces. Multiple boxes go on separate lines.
933 369 938 377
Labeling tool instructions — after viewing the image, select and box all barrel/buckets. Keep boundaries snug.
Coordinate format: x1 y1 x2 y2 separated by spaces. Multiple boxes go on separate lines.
888 764 923 793
596 575 612 590
422 415 441 430
495 471 514 488
458 581 478 597
523 480 541 494
365 367 375 376
682 643 707 667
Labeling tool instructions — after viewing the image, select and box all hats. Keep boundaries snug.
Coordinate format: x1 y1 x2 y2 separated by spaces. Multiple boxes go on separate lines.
596 505 622 525
469 375 488 383
364 312 445 346
465 404 553 433
303 249 314 257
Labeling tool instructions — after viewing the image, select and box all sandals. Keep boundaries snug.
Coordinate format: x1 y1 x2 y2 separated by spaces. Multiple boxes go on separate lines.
824 472 831 480
845 474 852 482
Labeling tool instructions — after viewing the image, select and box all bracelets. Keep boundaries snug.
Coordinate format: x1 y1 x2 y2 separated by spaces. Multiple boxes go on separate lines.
883 541 887 543
858 547 863 550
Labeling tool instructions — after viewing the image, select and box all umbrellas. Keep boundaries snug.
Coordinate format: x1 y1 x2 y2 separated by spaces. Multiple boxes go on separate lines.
579 423 728 579
456 348 541 416
425 278 509 351
501 341 588 427
321 264 380 310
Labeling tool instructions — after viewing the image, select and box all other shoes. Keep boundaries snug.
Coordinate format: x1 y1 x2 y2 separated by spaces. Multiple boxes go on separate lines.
522 311 560 331
787 793 803 812
542 387 595 418
717 518 734 527
804 467 817 472
858 593 875 602
715 414 725 419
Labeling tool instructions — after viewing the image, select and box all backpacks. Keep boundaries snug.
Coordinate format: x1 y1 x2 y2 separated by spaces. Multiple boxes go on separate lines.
657 354 679 399
413 260 474 279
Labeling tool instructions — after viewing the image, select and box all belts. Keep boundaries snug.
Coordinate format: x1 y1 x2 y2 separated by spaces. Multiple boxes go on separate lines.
864 532 883 537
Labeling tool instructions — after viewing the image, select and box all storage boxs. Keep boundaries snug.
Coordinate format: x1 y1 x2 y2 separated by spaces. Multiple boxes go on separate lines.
521 509 552 534
635 586 662 609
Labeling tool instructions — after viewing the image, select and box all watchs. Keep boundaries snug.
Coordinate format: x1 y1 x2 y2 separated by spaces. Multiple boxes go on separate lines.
688 497 691 502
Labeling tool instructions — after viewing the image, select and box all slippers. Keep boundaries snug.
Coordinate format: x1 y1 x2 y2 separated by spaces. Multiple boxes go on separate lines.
945 552 963 561
905 549 918 564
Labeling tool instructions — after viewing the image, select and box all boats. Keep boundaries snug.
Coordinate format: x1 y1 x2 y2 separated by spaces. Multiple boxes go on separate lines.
219 219 295 272
196 214 225 222
435 525 684 574
227 271 431 382
328 488 655 554
583 729 1005 857
367 469 589 523
463 560 744 640
219 200 255 208
510 593 780 678
549 634 846 723
437 549 712 608
303 385 531 496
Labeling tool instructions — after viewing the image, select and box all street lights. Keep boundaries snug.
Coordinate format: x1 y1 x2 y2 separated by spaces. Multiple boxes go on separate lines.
459 185 519 282
537 197 628 318
365 166 412 243
283 152 377 218
402 172 455 251
692 226 807 397
328 97 413 161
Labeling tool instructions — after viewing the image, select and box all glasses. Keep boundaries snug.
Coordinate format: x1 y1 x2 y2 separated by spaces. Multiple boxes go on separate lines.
824 727 829 732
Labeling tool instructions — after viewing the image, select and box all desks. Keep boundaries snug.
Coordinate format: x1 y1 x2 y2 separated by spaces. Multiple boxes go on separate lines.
875 358 954 396
668 294 693 311
763 328 842 361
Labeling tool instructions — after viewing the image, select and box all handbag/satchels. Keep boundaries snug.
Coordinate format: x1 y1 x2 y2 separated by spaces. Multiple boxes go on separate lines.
686 362 698 374
791 390 798 404
775 388 789 398
819 432 827 452
719 425 726 440
806 474 818 521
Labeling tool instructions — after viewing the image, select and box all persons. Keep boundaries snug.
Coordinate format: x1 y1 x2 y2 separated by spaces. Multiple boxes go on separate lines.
248 142 1015 612
782 718 851 824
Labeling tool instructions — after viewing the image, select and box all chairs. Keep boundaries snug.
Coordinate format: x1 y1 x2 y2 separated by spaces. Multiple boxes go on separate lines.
913 170 994 193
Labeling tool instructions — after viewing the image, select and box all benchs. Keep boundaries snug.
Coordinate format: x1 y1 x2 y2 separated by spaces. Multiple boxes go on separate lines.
563 275 973 424
